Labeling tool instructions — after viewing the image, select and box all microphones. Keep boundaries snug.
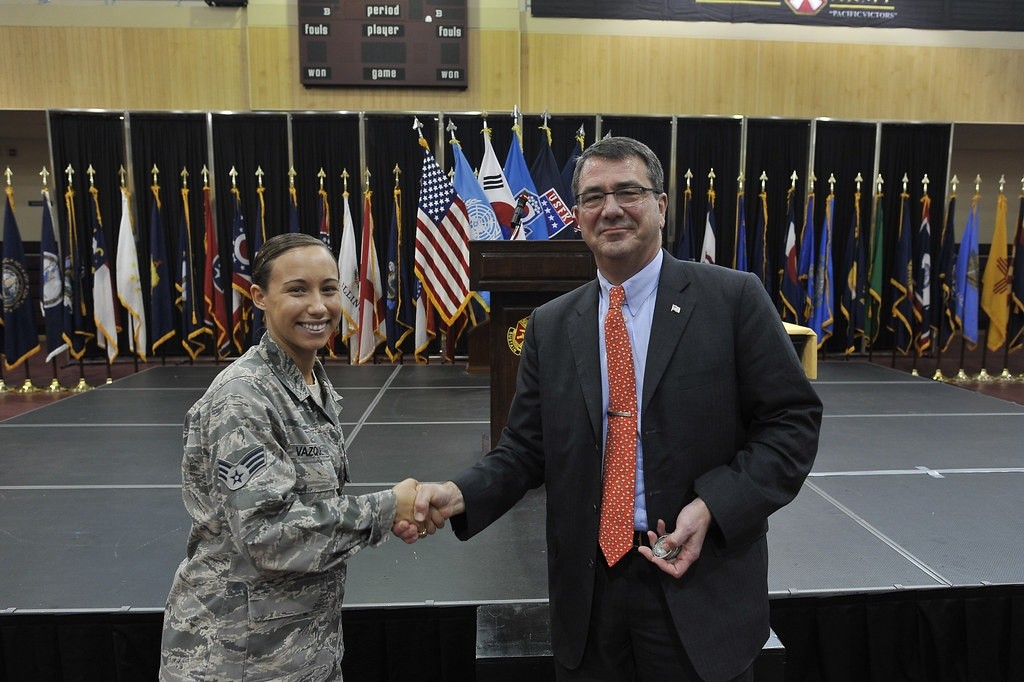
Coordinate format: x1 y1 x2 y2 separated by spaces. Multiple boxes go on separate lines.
510 193 528 227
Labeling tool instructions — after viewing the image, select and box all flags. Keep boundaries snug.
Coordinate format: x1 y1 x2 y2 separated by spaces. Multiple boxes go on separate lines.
0 190 412 372
412 125 586 366
672 188 1024 357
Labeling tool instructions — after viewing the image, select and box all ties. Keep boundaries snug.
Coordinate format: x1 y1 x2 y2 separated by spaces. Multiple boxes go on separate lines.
598 286 638 567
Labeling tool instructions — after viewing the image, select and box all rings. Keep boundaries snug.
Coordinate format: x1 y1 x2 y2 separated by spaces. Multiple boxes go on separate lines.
418 529 426 536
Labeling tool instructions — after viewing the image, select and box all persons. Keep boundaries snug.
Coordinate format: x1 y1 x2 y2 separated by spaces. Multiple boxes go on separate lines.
391 137 823 682
159 233 446 682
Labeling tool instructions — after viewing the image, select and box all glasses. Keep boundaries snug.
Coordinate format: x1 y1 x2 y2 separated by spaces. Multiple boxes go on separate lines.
576 186 660 212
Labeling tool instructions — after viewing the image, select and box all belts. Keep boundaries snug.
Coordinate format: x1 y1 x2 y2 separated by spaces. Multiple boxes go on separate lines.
634 530 651 548
